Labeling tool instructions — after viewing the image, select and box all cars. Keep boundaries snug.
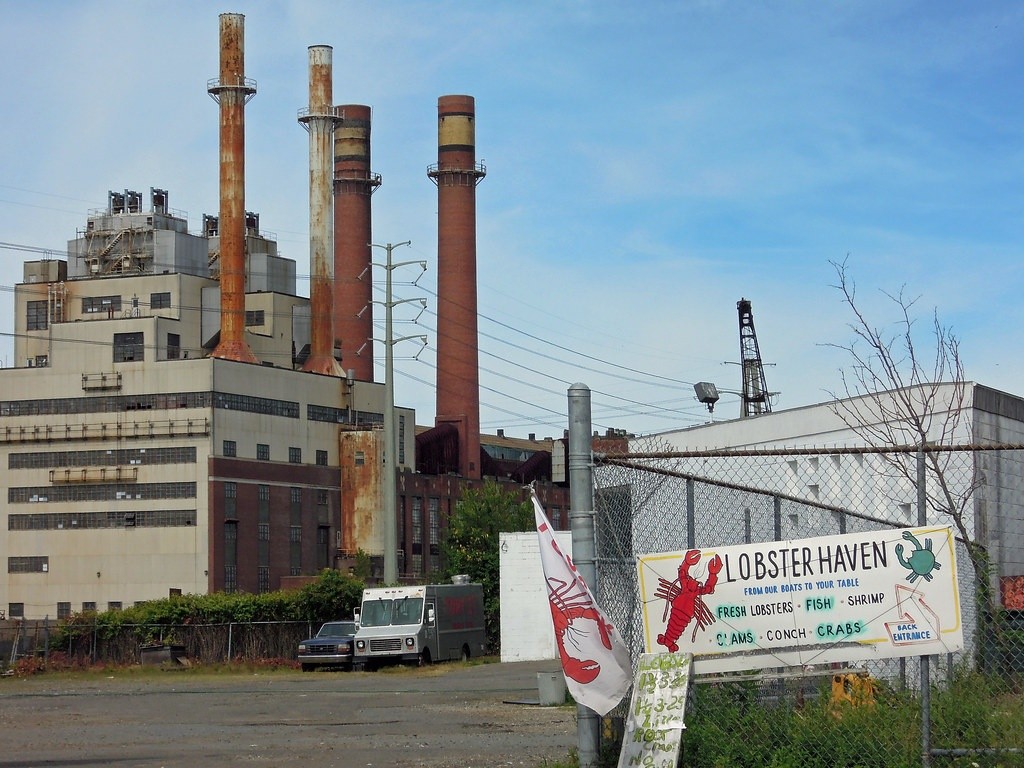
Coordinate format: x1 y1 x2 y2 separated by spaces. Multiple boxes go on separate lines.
298 622 357 672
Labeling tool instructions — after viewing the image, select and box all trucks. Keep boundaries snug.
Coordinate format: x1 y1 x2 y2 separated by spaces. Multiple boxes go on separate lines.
353 574 486 672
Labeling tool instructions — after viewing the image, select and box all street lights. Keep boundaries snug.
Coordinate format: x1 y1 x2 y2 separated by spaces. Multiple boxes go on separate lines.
693 382 720 424
